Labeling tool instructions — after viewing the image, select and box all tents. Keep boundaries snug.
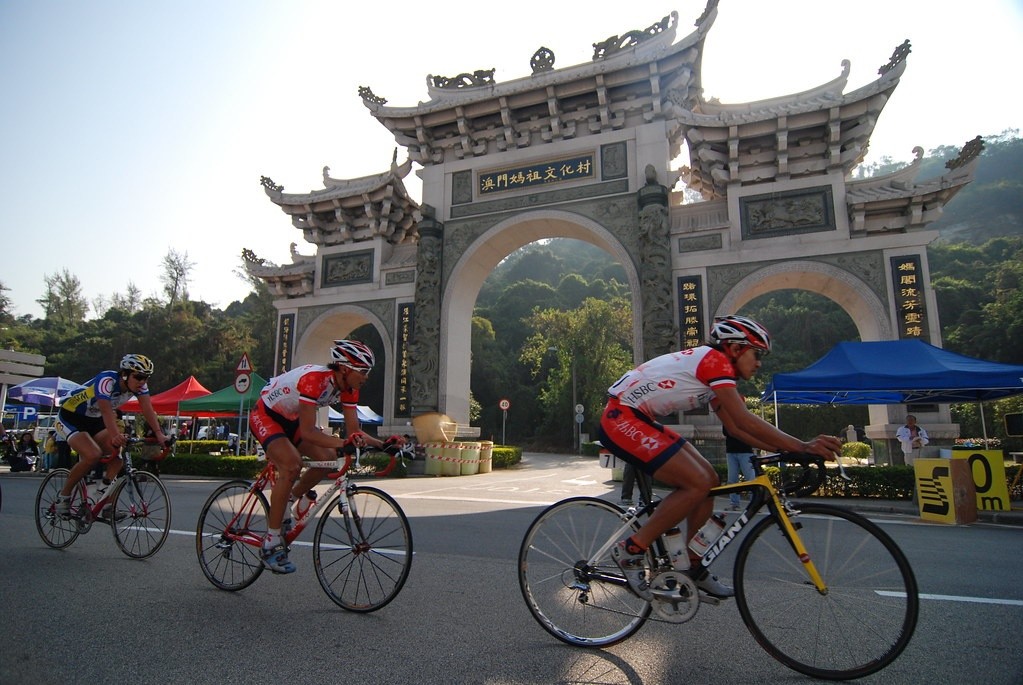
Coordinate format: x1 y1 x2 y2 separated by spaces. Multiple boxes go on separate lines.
340 405 383 429
759 337 1023 468
328 406 346 422
118 373 241 456
172 371 270 457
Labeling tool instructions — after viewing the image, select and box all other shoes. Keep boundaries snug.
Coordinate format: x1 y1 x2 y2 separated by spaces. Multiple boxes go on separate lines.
622 498 632 504
41 469 49 473
726 504 740 512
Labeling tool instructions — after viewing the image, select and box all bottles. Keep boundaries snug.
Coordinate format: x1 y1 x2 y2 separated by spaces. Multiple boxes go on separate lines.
280 497 291 524
661 525 691 572
687 510 729 557
86 480 97 504
290 489 318 520
97 478 110 496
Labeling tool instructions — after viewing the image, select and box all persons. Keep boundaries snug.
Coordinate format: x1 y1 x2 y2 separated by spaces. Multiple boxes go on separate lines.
333 426 340 438
399 434 416 459
53 354 171 523
721 423 761 513
210 421 231 440
41 431 73 474
597 316 843 601
136 412 165 482
615 462 653 509
249 337 386 576
180 423 188 435
112 408 125 433
9 432 39 472
896 415 930 467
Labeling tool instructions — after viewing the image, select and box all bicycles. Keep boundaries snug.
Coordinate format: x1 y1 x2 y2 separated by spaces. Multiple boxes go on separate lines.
518 446 920 680
196 432 414 614
35 434 178 560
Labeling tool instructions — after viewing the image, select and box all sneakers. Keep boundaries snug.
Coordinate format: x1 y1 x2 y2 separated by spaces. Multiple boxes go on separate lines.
55 491 72 521
611 540 654 603
696 570 735 598
102 507 127 521
259 543 296 573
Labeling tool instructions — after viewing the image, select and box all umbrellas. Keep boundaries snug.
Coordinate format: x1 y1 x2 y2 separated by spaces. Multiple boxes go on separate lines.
8 377 82 447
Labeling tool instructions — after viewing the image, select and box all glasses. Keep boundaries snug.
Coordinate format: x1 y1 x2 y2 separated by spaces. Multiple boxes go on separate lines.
339 360 372 376
743 346 762 361
127 372 151 382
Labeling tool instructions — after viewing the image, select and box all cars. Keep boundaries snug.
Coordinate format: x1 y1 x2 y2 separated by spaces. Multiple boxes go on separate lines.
196 425 239 441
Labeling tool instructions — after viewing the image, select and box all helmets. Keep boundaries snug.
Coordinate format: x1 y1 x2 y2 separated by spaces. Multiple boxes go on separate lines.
709 314 772 354
330 337 375 367
119 354 154 375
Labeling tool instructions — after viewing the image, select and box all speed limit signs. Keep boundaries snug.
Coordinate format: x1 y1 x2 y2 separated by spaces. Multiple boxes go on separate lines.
499 399 509 410
